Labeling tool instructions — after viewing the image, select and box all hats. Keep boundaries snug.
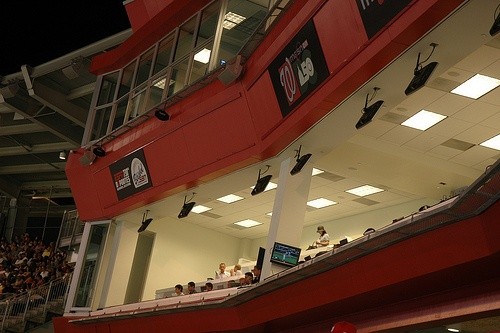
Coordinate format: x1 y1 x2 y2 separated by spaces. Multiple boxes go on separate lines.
316 225 325 232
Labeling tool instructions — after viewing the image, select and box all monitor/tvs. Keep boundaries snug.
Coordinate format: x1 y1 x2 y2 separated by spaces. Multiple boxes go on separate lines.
178 202 196 218
137 219 153 232
251 175 272 195
270 242 301 267
489 13 500 38
291 154 312 175
405 62 438 96
355 101 384 129
257 247 265 270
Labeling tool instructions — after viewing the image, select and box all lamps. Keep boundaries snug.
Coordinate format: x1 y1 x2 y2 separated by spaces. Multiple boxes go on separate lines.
60 150 66 160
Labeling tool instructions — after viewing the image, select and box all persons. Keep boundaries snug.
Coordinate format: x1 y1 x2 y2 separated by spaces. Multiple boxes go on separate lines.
245 271 253 284
230 264 243 276
315 225 329 247
0 232 76 313
188 281 200 294
228 280 235 287
239 277 245 285
214 262 230 278
252 266 261 282
174 284 184 295
205 282 213 290
